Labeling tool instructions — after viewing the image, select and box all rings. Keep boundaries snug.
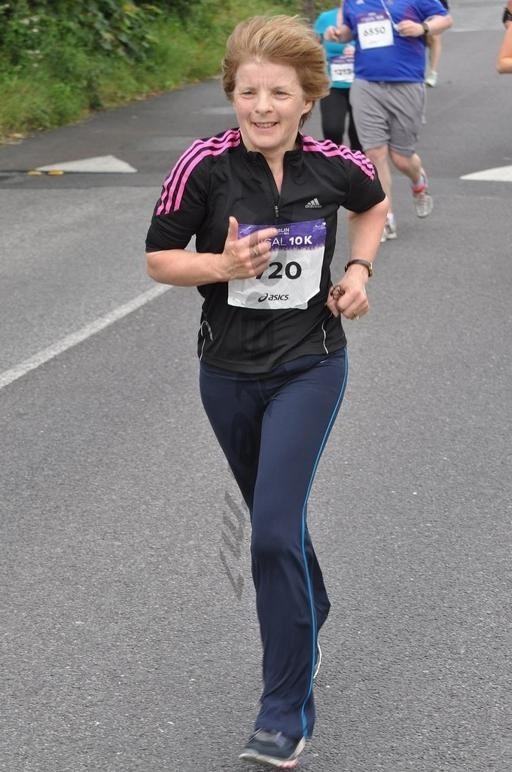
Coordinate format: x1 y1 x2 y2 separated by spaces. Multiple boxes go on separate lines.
352 311 359 321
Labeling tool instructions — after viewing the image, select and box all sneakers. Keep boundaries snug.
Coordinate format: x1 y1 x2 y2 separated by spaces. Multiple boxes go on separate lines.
380 213 398 243
410 166 433 218
238 727 306 768
424 72 438 88
312 640 322 680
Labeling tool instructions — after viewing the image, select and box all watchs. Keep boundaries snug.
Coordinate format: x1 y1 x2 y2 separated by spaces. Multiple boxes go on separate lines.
344 258 374 277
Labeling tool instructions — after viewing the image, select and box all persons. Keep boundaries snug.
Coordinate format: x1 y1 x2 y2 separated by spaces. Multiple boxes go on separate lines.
306 0 454 242
495 0 512 74
143 12 389 769
